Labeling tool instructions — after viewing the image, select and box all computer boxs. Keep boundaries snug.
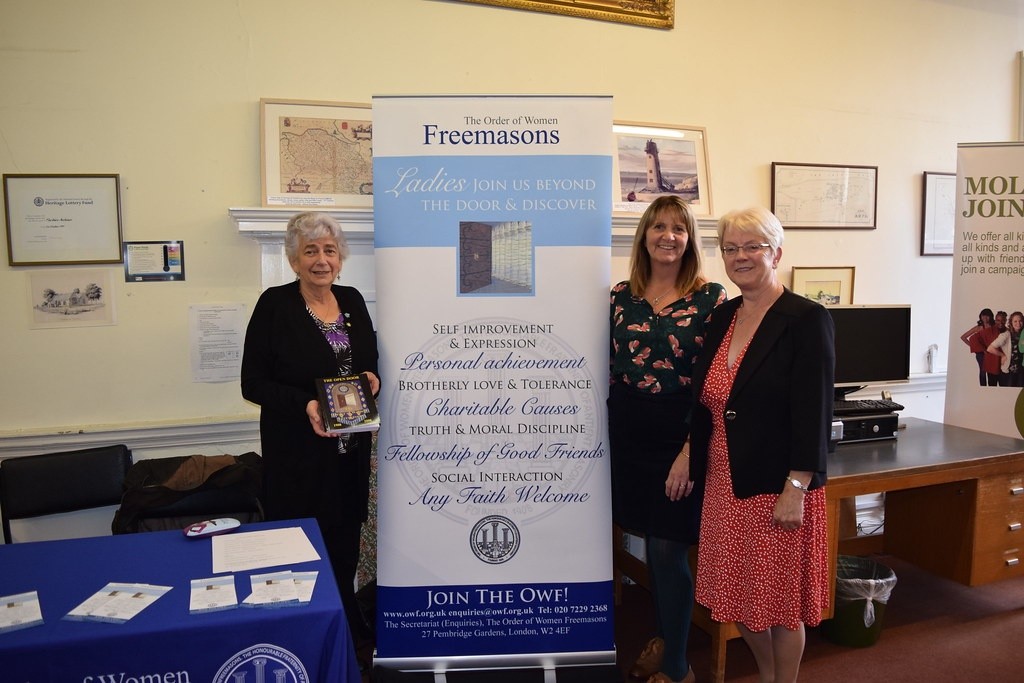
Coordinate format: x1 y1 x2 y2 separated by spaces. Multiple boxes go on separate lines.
829 412 899 444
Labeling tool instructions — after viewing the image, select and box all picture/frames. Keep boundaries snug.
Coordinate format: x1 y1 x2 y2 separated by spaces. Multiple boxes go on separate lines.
770 161 878 230
920 171 957 256
259 98 374 210
612 119 714 217
790 266 855 305
431 1 676 29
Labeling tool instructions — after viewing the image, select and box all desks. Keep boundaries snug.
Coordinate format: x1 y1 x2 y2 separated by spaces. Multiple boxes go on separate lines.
613 418 1024 683
0 518 363 683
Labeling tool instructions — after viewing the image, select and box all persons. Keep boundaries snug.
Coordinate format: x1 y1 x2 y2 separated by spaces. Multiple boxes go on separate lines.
241 214 382 675
969 311 1009 386
686 205 835 683
960 308 995 386
608 194 730 683
987 312 1024 388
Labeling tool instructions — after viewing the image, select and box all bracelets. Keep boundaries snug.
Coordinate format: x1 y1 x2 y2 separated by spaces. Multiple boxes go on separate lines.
681 451 690 458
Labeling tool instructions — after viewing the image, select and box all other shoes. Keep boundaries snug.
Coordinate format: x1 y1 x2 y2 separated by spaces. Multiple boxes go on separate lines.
356 656 370 673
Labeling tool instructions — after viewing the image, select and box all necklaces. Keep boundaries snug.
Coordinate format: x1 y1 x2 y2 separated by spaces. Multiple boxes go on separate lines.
647 283 674 304
737 303 767 324
305 295 330 329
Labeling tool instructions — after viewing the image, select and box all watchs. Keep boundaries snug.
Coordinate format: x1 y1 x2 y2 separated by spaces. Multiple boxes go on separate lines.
786 476 809 494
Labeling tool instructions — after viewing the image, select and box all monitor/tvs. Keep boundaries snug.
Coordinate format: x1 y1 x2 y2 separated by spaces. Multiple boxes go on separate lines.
825 304 910 400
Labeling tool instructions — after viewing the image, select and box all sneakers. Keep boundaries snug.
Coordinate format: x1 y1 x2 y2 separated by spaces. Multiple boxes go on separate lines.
646 663 695 683
627 636 666 682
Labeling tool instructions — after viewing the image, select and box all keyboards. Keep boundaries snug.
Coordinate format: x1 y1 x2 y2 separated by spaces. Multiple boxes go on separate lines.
833 399 905 416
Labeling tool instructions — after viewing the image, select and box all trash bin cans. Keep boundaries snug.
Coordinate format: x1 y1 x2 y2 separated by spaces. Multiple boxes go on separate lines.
821 555 896 646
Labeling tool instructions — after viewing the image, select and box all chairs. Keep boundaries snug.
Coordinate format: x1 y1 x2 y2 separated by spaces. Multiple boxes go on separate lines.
0 443 132 545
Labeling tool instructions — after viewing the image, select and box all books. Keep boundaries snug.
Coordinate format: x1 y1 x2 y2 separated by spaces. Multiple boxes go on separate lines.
314 373 381 435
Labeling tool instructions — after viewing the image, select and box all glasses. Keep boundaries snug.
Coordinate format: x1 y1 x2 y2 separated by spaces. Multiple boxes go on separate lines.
721 243 770 255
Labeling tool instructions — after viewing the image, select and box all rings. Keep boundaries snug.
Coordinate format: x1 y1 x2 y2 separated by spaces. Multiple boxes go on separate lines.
680 484 685 489
